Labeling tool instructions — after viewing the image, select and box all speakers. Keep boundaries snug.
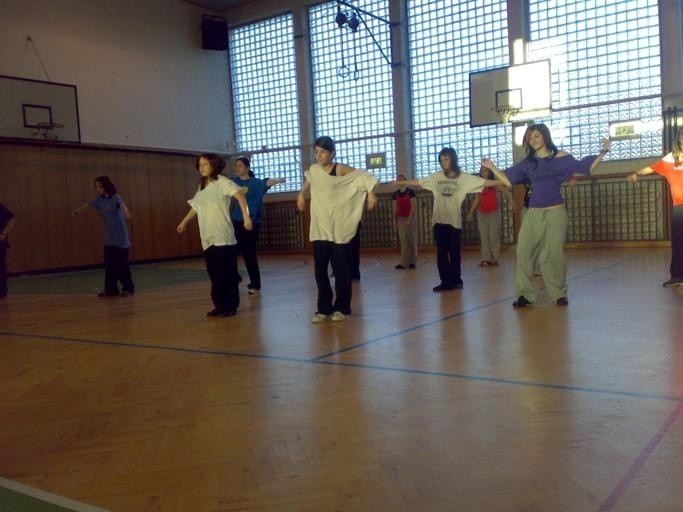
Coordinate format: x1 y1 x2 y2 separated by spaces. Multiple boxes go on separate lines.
202 18 229 51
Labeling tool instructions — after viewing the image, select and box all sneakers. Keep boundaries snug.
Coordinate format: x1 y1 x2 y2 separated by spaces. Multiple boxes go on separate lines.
97 287 260 318
662 277 683 287
332 311 352 322
557 297 569 305
512 296 531 308
311 312 332 323
396 261 500 292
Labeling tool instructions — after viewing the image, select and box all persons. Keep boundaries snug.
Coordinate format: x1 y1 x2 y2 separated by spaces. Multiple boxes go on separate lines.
521 181 542 276
0 206 19 300
478 124 612 307
227 158 287 294
625 124 683 287
329 220 360 281
465 164 518 268
392 175 418 269
177 153 252 319
389 147 506 292
71 176 134 298
296 136 380 324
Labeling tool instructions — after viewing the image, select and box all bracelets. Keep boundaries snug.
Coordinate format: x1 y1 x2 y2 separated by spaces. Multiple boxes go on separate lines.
73 210 78 215
633 172 641 178
599 150 609 156
1 232 8 237
